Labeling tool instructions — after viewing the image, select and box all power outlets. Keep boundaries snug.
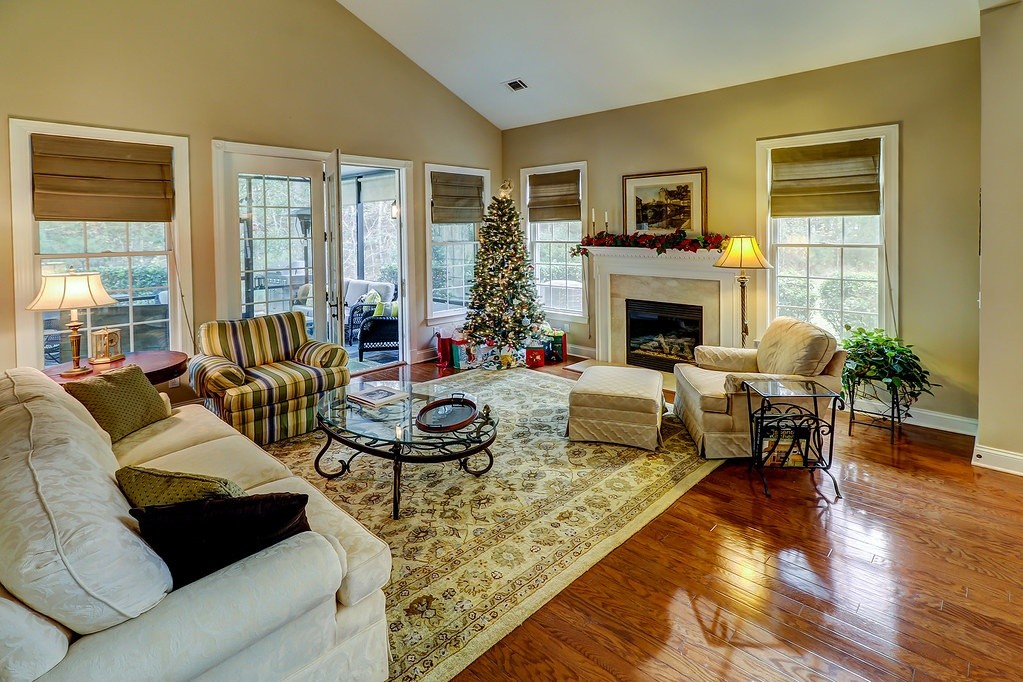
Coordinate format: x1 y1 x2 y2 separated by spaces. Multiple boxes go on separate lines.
169 377 180 389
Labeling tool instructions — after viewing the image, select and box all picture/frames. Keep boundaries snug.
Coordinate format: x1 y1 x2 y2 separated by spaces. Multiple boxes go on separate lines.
622 168 709 239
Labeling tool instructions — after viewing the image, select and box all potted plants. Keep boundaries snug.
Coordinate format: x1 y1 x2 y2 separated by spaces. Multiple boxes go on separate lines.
836 322 944 418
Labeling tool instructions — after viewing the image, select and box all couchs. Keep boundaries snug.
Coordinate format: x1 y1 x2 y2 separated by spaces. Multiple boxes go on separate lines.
673 316 848 459
0 366 392 682
186 277 395 446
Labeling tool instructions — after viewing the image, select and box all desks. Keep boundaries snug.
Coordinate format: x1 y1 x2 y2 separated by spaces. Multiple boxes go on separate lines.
41 351 188 385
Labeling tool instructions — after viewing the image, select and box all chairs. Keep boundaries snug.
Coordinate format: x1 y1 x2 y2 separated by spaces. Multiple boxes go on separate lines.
43 319 61 364
348 302 399 362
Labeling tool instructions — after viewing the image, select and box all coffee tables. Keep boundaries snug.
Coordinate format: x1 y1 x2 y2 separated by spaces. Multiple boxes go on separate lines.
742 380 846 499
314 380 499 520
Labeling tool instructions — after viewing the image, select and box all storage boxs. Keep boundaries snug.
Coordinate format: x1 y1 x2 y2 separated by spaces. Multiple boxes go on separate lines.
525 332 568 366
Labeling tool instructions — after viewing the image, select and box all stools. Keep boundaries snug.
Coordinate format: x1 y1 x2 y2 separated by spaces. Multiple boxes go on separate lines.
564 365 664 451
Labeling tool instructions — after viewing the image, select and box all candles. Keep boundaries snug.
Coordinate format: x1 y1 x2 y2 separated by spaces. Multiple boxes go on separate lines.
591 206 595 222
604 209 608 222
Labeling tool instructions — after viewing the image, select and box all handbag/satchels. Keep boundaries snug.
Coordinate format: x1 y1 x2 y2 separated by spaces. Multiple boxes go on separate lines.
452 337 471 369
526 340 545 368
542 328 567 363
437 337 452 368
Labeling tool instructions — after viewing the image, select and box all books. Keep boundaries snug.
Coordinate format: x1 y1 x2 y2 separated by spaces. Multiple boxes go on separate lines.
347 385 409 407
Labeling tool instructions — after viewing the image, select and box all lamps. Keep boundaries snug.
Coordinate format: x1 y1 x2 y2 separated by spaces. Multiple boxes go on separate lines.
391 200 398 219
24 265 119 377
713 234 774 348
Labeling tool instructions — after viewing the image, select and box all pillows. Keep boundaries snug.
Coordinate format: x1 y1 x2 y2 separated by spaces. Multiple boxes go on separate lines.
58 364 169 443
115 464 312 593
305 284 328 308
353 289 398 317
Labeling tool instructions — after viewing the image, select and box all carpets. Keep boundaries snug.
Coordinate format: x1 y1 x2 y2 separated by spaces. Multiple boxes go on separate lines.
258 367 726 682
